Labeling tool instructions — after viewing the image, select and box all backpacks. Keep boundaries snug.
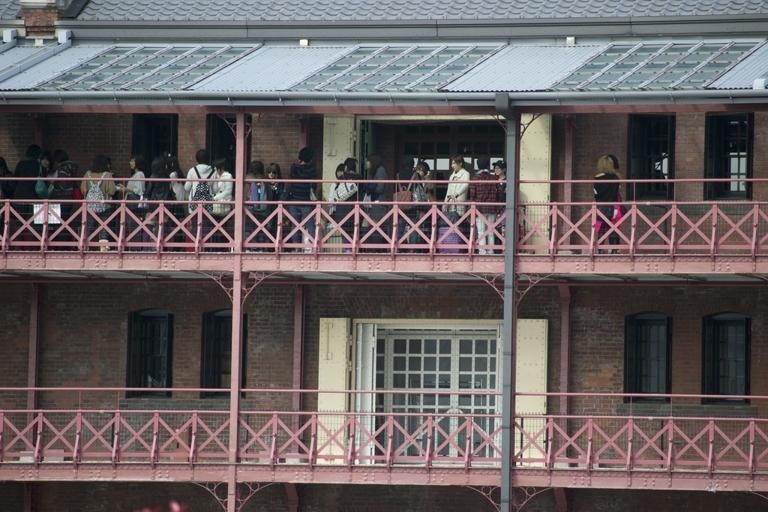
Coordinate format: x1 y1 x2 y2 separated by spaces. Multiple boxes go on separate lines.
86 170 108 214
246 175 268 212
190 164 216 213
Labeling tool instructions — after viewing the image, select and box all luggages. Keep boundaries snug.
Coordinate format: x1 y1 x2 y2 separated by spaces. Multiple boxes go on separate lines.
439 224 463 253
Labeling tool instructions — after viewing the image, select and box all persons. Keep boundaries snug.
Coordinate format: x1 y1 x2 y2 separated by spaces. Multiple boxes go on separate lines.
1 145 236 256
592 154 619 255
245 145 506 255
606 155 627 254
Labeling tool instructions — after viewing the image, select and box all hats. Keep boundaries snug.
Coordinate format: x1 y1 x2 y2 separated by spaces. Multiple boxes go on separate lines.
492 160 506 169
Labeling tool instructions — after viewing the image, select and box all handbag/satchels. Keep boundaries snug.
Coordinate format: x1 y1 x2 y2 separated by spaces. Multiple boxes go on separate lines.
413 189 428 203
72 187 83 206
333 180 357 202
393 189 412 210
438 210 464 226
35 180 48 198
